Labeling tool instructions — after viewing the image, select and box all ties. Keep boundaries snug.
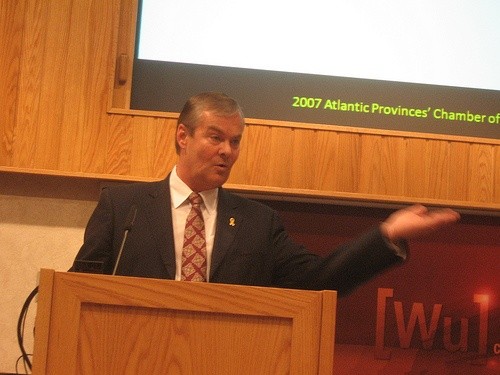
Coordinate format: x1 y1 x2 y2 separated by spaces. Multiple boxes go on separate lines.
180 194 208 282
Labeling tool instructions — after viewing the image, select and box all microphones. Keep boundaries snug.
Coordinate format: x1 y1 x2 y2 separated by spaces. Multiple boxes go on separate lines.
112 205 139 276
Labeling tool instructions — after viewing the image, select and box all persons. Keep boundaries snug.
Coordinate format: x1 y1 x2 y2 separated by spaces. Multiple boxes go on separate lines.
68 92 460 297
